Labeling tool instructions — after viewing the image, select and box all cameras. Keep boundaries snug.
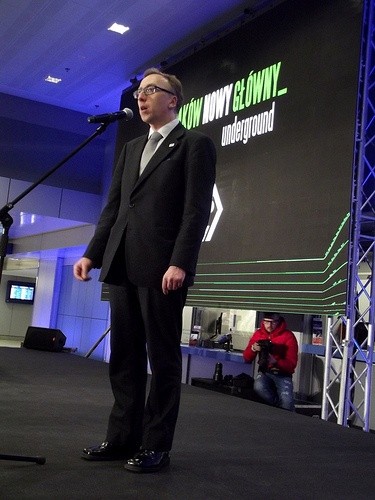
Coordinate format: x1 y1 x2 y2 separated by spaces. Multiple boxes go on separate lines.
257 340 271 351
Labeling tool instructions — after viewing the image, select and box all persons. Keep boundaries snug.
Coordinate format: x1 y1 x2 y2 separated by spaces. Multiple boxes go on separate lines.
243 312 299 413
73 68 216 472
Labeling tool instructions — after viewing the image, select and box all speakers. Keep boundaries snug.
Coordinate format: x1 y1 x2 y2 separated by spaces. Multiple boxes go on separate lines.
23 325 67 353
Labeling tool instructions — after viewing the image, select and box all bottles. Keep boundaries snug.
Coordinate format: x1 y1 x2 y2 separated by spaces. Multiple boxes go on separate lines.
189 325 201 347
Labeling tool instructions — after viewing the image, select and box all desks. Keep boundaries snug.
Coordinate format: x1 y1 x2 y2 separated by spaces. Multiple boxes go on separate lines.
302 343 375 367
180 343 251 384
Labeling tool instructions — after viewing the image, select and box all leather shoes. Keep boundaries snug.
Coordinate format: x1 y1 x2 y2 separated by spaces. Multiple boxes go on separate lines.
80 440 141 461
125 447 169 469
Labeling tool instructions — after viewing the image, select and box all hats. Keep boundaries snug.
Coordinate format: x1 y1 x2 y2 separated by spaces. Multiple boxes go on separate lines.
263 311 279 319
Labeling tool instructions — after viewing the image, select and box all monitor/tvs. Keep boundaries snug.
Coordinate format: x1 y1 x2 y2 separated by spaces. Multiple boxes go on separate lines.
5 280 35 304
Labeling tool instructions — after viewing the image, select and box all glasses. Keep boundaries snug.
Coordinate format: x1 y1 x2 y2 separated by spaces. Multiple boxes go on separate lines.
133 85 177 98
263 319 278 325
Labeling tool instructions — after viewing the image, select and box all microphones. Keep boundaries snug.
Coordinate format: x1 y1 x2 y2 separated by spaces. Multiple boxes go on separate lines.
87 108 134 123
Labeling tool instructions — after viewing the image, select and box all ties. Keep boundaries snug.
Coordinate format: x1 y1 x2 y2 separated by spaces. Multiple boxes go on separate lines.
137 132 163 178
